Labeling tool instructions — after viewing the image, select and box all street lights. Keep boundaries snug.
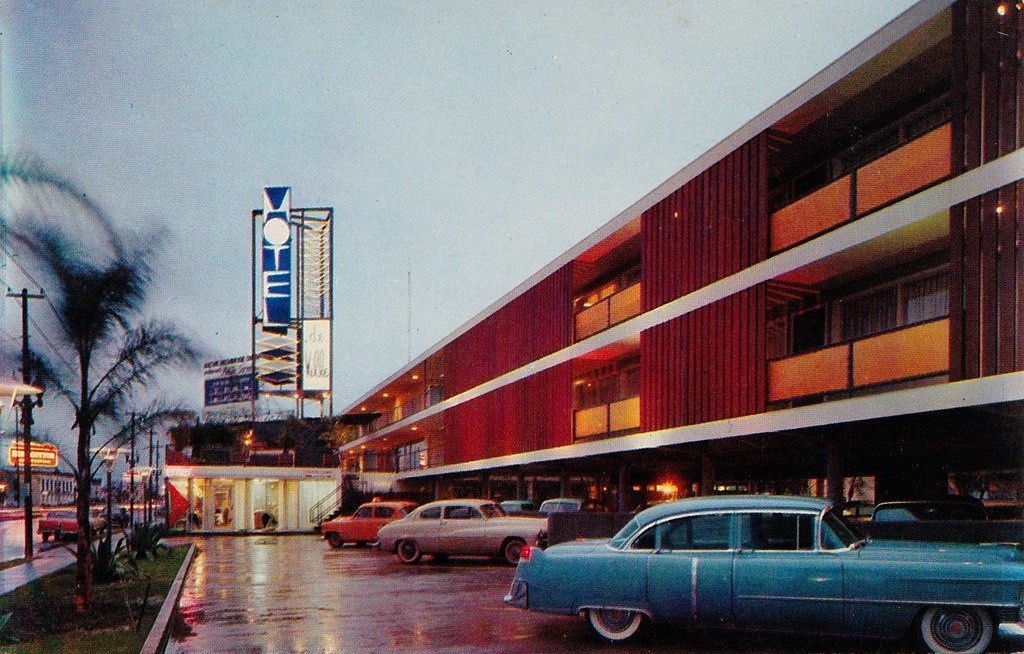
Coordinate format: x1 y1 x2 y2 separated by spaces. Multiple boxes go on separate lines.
127 466 157 526
123 446 141 532
8 369 46 557
89 447 133 558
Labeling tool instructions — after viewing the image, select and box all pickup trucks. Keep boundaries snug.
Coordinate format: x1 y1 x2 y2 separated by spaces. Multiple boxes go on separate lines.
375 499 549 567
321 500 420 548
504 493 1023 654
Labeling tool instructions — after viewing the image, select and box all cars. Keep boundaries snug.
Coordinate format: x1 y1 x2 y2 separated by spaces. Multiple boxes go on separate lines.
90 508 106 535
101 506 130 526
154 506 166 518
38 510 78 542
